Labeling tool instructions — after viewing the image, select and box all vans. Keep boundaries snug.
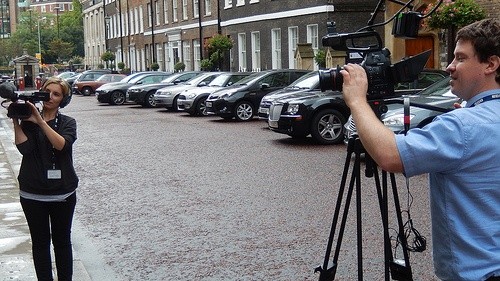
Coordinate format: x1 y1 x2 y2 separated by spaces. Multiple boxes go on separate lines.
206 70 312 122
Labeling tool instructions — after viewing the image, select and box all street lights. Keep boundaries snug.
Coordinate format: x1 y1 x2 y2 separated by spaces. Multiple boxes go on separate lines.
54 6 61 65
37 17 47 53
29 9 32 38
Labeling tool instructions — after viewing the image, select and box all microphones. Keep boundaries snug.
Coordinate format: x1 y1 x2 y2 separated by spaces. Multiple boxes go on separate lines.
0 82 16 98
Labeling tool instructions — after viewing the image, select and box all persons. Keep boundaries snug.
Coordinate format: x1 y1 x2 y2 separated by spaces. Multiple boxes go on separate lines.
339 17 500 281
12 77 79 281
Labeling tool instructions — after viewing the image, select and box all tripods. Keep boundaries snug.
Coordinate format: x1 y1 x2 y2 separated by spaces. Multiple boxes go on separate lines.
314 135 414 281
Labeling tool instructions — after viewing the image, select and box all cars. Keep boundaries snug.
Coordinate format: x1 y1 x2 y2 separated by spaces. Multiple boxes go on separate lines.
342 74 467 147
56 71 82 95
267 69 449 145
95 72 173 105
257 67 341 122
75 73 128 96
153 73 223 112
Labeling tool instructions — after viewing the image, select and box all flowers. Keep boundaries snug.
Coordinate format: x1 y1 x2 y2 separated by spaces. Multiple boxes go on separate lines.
420 0 485 32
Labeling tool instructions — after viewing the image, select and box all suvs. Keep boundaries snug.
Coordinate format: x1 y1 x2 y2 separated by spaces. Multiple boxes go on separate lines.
177 73 251 117
126 71 203 108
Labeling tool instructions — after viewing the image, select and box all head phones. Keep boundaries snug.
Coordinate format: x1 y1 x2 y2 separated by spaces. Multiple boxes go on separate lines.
39 79 72 108
